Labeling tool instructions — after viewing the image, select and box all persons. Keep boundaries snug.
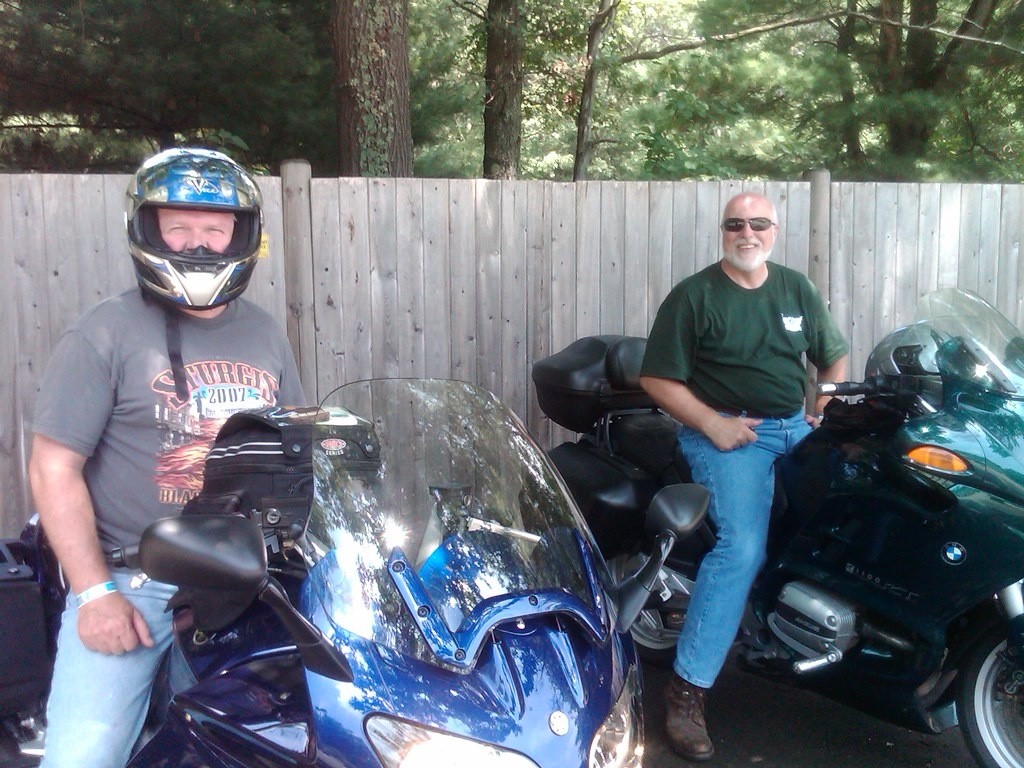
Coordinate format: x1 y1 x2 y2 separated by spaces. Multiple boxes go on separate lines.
29 146 309 768
638 192 853 762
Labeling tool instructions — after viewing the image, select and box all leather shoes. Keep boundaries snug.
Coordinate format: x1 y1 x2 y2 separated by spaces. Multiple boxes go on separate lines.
660 670 715 762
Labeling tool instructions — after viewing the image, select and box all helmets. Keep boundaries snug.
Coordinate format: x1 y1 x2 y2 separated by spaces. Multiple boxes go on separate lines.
863 323 953 408
122 147 265 312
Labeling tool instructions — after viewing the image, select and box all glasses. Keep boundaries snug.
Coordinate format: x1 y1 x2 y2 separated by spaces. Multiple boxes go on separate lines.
720 217 778 232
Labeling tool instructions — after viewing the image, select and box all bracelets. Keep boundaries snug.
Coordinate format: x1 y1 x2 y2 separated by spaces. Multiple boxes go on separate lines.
814 412 825 419
75 581 119 610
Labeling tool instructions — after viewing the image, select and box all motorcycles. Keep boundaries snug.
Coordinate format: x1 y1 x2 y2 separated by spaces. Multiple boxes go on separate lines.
0 374 645 768
519 285 1024 768
